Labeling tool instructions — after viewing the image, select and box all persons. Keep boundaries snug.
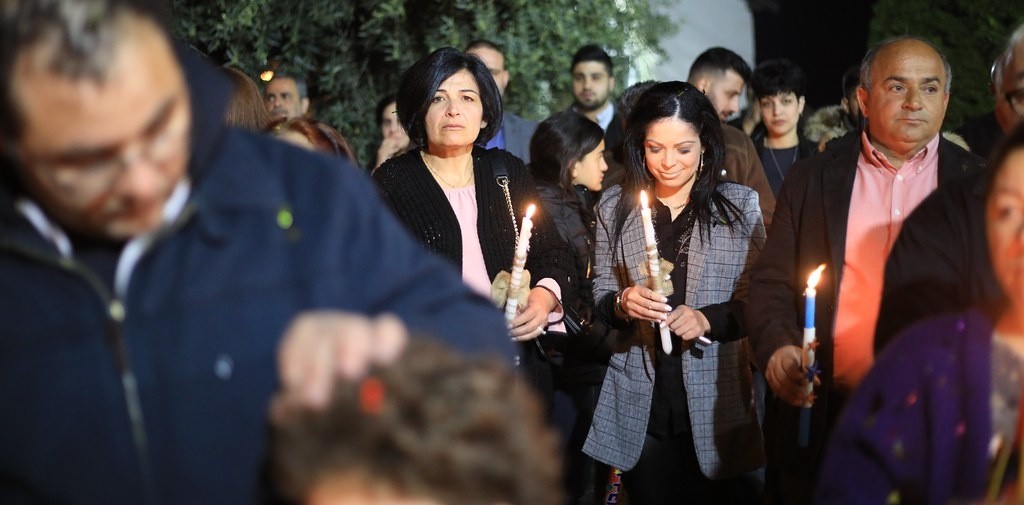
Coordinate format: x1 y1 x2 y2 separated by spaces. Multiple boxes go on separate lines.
221 39 864 505
260 340 561 504
824 116 1024 505
0 0 514 505
744 34 986 505
873 20 1024 358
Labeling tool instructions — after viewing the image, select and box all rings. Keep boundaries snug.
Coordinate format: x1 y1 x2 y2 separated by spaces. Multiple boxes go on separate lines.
538 327 546 335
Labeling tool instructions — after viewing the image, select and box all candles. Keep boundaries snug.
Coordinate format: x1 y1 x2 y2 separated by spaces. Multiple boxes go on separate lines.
505 204 535 320
640 189 673 355
799 264 826 448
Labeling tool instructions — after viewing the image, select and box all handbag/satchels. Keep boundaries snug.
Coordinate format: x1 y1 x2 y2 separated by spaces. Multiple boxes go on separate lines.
563 276 600 338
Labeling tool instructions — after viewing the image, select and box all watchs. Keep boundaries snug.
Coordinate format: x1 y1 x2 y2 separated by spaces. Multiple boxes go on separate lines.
615 287 630 316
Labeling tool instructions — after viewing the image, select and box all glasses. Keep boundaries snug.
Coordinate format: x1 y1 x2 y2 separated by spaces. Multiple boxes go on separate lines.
993 87 1024 116
